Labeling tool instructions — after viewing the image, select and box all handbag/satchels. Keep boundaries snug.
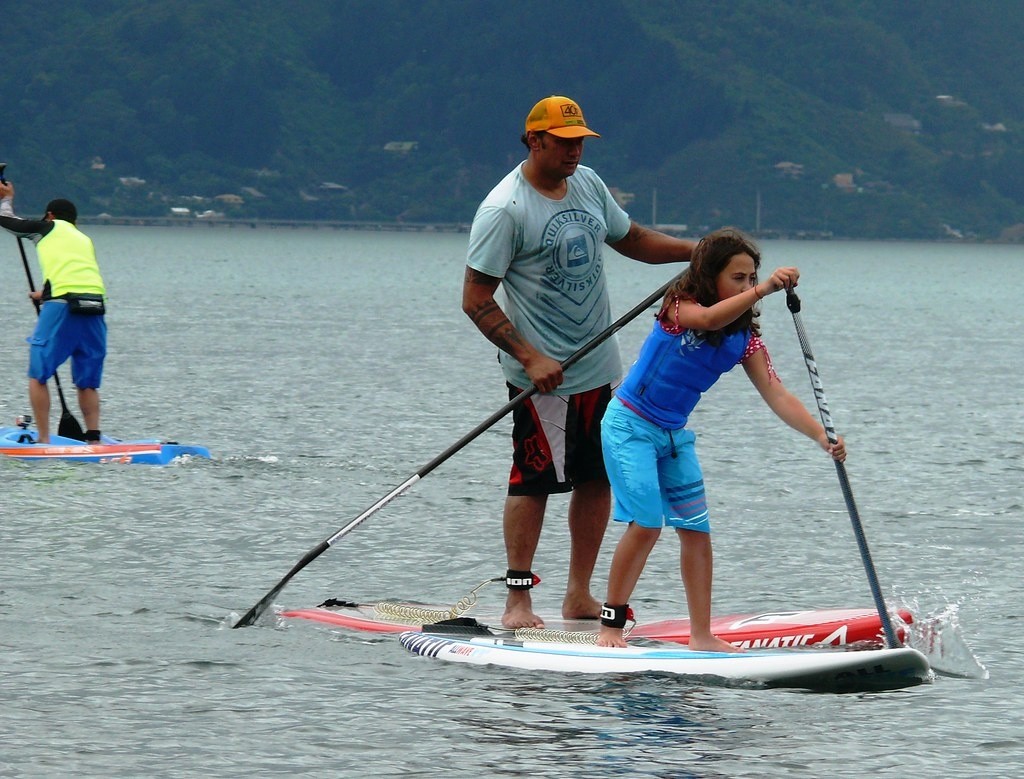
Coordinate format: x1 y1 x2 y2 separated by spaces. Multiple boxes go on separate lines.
65 292 105 315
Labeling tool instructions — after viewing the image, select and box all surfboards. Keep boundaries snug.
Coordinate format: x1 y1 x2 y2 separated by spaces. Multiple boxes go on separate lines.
0 424 211 467
397 625 936 697
277 596 913 652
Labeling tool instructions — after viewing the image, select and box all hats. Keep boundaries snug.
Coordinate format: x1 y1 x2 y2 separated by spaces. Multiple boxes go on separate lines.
525 95 601 138
40 199 78 221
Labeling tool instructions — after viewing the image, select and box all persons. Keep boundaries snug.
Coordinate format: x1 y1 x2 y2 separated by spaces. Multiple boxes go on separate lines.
463 94 697 630
0 180 107 445
595 236 845 652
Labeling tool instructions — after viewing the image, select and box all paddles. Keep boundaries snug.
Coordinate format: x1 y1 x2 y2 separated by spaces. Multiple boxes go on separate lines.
774 279 905 650
232 266 690 630
0 160 87 442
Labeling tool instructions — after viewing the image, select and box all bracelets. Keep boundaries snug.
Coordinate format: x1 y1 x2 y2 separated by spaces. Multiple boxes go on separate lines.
755 286 763 299
